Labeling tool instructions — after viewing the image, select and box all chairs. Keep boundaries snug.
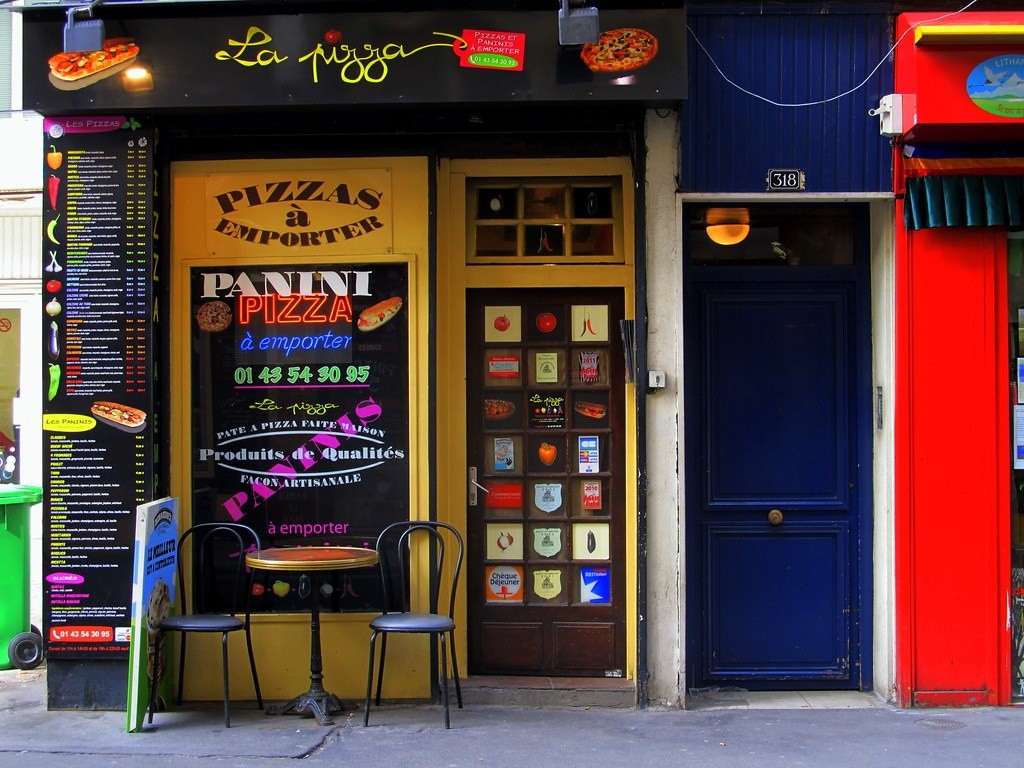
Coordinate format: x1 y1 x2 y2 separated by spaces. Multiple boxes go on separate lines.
362 520 464 730
148 521 265 727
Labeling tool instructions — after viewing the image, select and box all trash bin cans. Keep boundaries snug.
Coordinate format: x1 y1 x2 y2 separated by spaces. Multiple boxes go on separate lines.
0 485 44 671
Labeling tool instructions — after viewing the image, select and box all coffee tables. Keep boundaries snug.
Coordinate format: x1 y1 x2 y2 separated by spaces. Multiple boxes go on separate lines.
245 545 381 727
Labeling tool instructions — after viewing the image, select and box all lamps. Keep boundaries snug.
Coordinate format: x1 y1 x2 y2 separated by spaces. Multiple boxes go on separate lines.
557 7 600 45
64 19 106 54
705 219 751 247
